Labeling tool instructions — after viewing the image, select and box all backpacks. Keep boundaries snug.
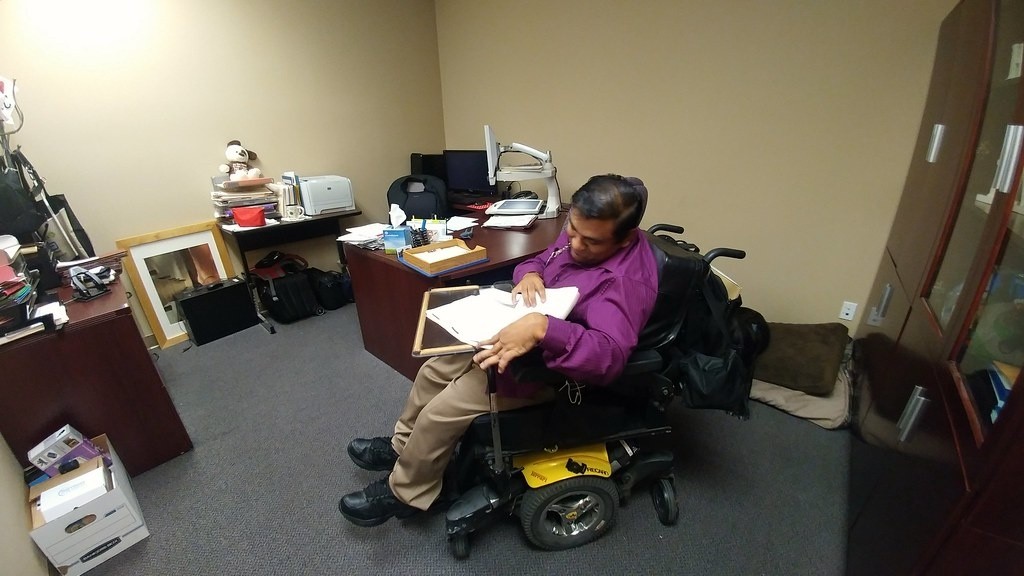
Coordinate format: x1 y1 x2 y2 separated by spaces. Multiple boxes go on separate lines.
670 237 771 423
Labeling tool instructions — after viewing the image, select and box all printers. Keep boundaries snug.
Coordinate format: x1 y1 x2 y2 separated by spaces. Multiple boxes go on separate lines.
300 175 356 216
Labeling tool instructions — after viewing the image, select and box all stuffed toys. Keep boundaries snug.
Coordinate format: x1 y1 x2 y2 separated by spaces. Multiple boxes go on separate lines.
219 140 261 182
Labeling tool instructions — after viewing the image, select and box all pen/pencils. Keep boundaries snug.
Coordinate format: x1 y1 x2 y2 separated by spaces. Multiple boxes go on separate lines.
410 226 415 232
422 219 426 230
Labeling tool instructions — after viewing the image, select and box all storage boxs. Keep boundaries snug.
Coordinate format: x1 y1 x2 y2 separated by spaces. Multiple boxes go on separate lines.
403 238 486 275
23 423 150 576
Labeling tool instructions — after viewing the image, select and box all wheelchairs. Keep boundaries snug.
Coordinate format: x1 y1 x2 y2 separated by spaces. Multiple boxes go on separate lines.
435 222 748 562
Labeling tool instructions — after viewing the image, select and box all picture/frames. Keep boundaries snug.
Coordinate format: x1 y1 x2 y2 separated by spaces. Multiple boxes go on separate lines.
117 220 236 350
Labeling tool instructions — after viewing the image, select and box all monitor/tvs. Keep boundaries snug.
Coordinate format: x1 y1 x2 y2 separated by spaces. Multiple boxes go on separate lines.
411 124 544 214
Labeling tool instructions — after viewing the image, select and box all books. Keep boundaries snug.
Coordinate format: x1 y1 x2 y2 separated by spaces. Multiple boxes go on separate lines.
271 175 301 218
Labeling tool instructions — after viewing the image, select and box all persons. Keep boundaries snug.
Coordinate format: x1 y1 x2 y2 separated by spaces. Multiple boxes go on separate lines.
338 175 660 528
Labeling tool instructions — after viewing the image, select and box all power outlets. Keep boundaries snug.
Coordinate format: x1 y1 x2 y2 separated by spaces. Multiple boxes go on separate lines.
839 300 857 320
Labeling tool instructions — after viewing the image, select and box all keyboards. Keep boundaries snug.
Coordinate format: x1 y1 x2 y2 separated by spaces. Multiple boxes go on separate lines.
448 197 477 205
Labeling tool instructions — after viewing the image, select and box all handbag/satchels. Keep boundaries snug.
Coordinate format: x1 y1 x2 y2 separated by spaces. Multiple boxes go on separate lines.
253 251 352 325
0 123 52 246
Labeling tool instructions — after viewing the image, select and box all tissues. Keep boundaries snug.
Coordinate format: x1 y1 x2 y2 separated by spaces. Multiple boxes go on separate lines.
382 203 415 255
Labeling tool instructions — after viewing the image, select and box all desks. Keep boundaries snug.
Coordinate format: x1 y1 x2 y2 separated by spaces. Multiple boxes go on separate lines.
344 205 571 382
214 203 363 334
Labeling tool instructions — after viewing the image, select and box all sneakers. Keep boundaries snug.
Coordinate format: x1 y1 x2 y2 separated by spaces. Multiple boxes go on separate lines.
348 437 401 472
338 471 421 527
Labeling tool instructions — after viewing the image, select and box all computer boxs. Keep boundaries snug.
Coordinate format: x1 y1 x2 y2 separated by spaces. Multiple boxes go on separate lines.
173 277 259 346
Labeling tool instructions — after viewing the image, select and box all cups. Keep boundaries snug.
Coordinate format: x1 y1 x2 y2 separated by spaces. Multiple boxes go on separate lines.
286 205 304 219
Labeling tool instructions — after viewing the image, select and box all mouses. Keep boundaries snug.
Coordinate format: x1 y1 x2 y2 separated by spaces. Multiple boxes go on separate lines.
475 201 485 206
207 283 223 290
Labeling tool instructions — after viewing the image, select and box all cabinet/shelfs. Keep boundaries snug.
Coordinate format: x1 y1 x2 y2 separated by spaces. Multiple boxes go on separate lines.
847 0 1024 576
0 273 194 476
